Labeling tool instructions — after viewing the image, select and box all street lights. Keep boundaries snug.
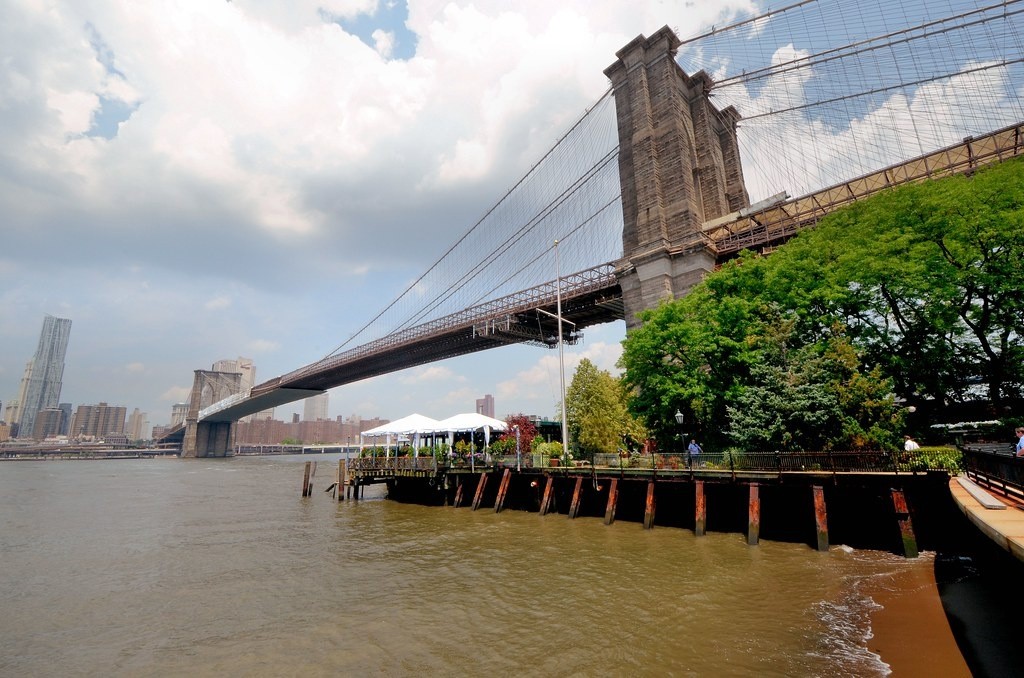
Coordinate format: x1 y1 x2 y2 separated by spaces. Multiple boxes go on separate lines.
675 409 689 469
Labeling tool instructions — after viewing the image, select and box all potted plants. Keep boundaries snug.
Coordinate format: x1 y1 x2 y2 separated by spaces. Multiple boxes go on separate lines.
357 435 563 468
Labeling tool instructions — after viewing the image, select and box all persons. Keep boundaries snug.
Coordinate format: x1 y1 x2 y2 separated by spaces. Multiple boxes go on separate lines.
904 435 921 476
1015 426 1024 488
688 439 704 469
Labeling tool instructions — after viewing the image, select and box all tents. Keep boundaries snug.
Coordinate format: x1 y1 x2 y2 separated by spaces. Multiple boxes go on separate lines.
359 413 521 473
